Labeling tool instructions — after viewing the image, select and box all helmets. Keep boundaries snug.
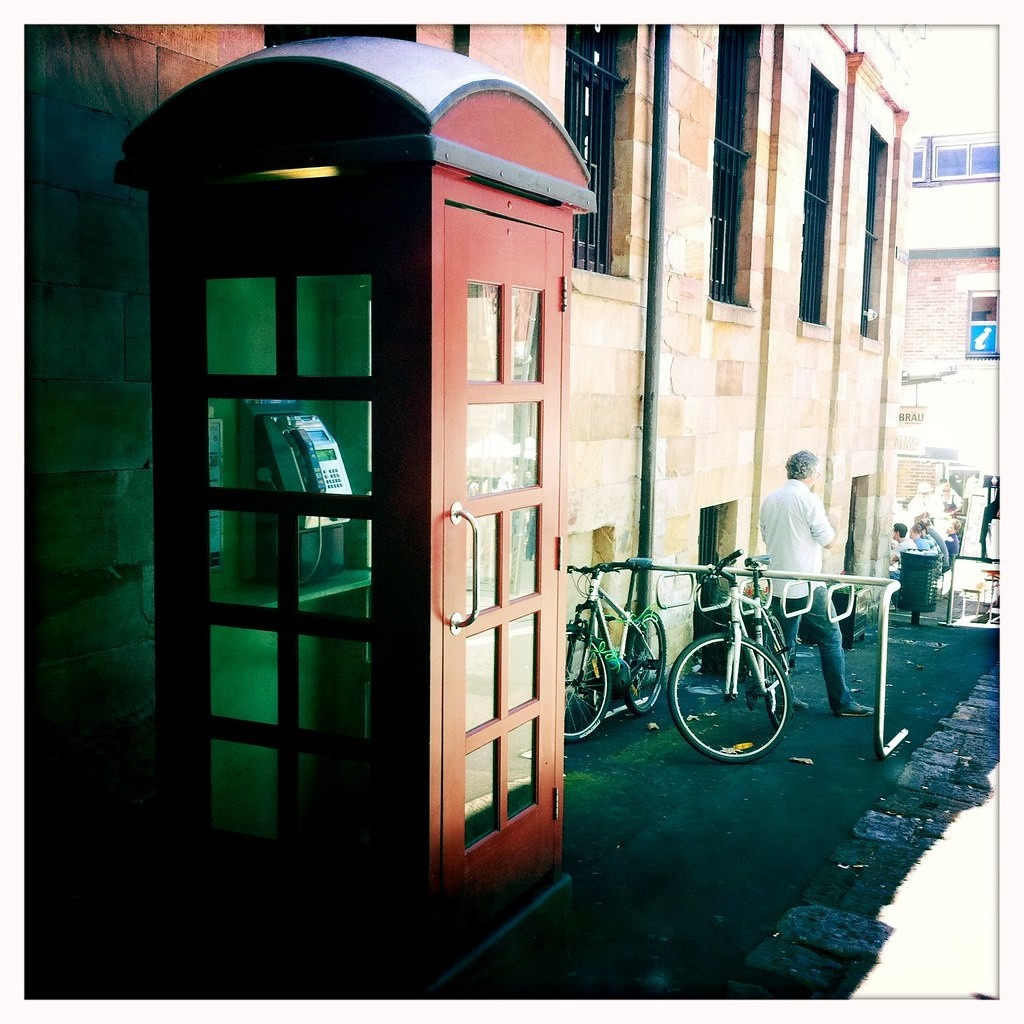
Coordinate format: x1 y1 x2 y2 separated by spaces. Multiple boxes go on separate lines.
610 658 631 701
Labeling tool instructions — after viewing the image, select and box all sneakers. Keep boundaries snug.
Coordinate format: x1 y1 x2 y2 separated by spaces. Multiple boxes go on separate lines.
777 696 810 710
833 702 874 716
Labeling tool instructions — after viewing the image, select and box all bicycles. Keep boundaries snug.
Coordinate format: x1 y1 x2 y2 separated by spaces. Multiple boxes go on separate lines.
666 550 794 765
564 558 666 743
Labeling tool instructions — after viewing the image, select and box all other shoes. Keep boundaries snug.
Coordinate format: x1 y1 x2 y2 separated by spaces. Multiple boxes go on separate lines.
889 604 896 610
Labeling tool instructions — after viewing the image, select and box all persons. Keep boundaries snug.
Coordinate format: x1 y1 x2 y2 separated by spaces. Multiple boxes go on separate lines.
759 449 875 718
889 518 961 611
942 482 963 519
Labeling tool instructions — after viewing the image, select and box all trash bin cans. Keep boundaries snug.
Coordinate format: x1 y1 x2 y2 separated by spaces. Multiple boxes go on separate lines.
897 548 939 613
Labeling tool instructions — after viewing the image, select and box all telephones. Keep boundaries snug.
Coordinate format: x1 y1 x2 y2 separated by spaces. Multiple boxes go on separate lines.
276 411 355 531
